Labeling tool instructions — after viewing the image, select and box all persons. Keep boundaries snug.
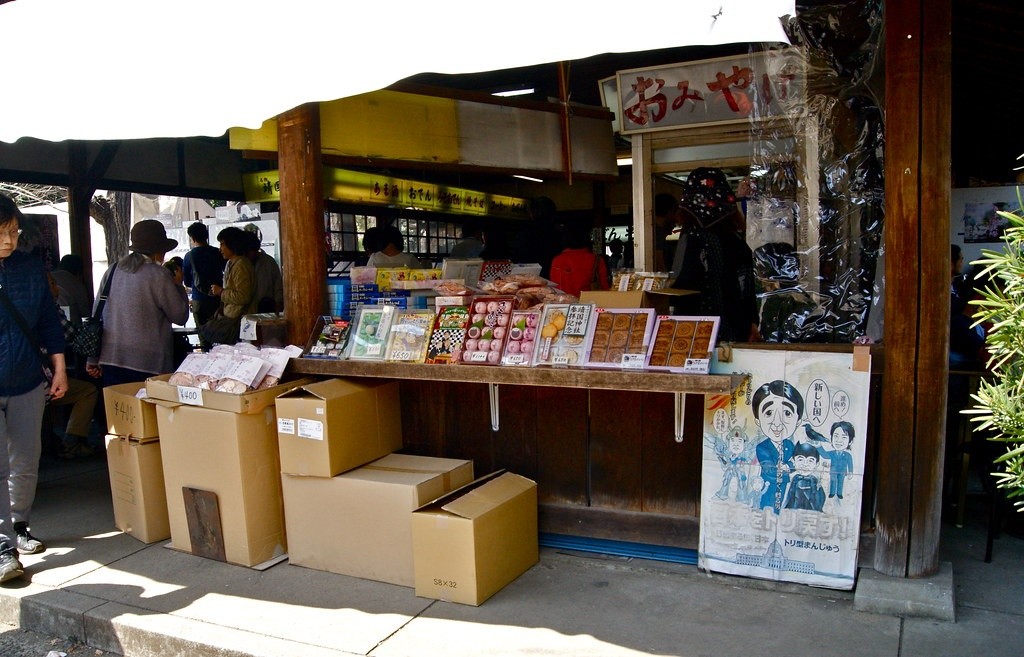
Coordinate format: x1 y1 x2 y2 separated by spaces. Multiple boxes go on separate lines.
362 225 424 268
950 244 1006 355
449 195 623 298
653 165 818 341
46 220 282 457
0 194 68 582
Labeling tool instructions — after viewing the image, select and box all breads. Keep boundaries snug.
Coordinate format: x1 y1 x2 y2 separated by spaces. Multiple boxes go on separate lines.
480 273 579 305
433 281 474 295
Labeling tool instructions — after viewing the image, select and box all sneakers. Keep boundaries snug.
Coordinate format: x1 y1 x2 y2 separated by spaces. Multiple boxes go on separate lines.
12 521 44 555
0 541 24 583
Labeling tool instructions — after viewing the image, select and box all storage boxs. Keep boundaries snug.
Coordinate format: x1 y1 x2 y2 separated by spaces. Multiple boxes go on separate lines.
102 258 721 607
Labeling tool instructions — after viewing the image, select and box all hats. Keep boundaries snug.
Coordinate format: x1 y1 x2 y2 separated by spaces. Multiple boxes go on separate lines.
128 219 178 255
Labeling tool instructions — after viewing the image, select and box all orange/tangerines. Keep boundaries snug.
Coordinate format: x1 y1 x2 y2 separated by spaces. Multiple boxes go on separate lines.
541 314 566 338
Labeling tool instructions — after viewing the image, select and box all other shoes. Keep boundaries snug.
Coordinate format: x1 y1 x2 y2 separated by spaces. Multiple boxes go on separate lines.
57 442 98 460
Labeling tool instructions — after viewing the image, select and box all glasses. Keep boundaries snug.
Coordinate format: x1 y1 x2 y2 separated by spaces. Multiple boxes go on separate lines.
0 229 23 235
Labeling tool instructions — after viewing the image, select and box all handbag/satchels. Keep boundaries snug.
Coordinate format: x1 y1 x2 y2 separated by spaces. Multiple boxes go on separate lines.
199 307 242 346
73 318 103 357
195 273 223 297
42 367 55 405
